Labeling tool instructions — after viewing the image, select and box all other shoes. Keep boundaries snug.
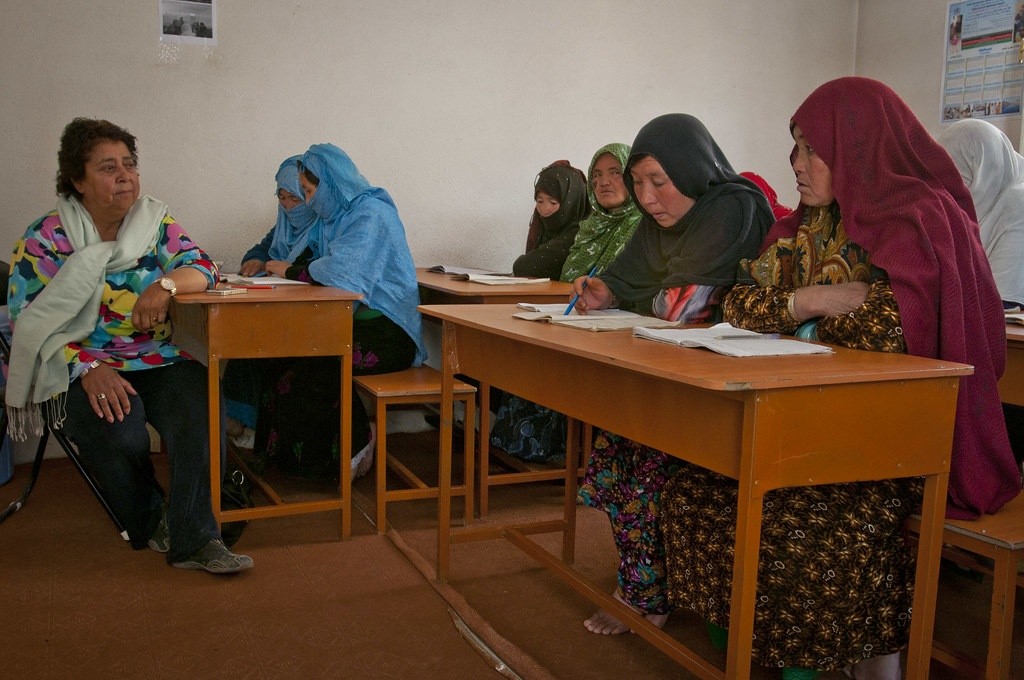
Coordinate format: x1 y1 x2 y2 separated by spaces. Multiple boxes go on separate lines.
349 421 375 484
424 415 464 434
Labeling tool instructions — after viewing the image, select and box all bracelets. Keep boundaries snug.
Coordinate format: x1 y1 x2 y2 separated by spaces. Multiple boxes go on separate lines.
795 322 816 340
788 292 803 323
79 359 103 379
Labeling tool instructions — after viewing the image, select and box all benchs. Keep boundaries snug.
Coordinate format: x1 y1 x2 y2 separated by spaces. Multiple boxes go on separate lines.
899 487 1024 680
353 358 477 537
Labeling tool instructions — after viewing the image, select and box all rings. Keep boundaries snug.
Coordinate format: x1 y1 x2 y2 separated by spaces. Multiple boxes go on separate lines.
153 317 158 322
98 393 106 399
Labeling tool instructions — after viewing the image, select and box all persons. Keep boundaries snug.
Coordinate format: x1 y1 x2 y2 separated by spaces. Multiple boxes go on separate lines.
463 113 794 637
8 116 253 574
937 117 1023 313
660 77 1022 680
225 143 428 491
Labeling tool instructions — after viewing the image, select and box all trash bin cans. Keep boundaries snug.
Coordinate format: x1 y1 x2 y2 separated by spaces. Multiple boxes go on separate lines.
0 321 15 484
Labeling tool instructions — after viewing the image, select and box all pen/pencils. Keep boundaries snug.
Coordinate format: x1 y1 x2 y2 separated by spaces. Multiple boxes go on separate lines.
227 286 275 289
713 334 779 339
563 266 598 315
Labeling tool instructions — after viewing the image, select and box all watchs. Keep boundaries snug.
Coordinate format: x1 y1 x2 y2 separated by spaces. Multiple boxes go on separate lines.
152 277 177 296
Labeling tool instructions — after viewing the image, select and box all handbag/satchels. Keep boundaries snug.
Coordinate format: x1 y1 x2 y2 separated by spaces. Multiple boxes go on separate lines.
220 470 255 547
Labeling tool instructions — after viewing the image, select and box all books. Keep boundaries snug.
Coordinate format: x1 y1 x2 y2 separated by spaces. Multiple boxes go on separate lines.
232 276 311 285
425 265 512 276
218 271 241 284
633 322 835 355
450 273 551 285
517 302 687 333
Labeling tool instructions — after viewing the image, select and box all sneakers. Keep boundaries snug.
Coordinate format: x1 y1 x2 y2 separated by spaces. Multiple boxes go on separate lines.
146 514 169 552
170 536 254 572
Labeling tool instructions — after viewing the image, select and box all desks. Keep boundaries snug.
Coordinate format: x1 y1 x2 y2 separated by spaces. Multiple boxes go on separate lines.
169 269 364 544
417 304 975 680
414 267 591 522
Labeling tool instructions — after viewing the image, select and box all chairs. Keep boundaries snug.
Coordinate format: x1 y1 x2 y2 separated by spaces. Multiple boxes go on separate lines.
0 258 164 541
